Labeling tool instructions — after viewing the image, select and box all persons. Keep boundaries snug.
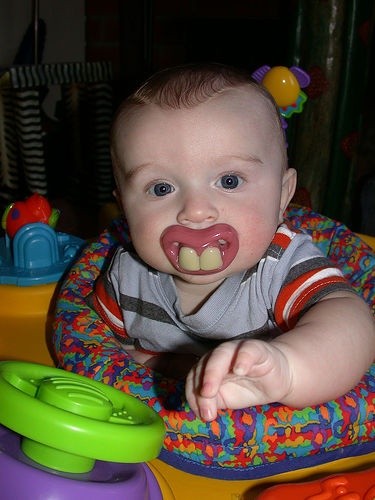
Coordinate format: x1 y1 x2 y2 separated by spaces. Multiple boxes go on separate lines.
84 62 375 421
